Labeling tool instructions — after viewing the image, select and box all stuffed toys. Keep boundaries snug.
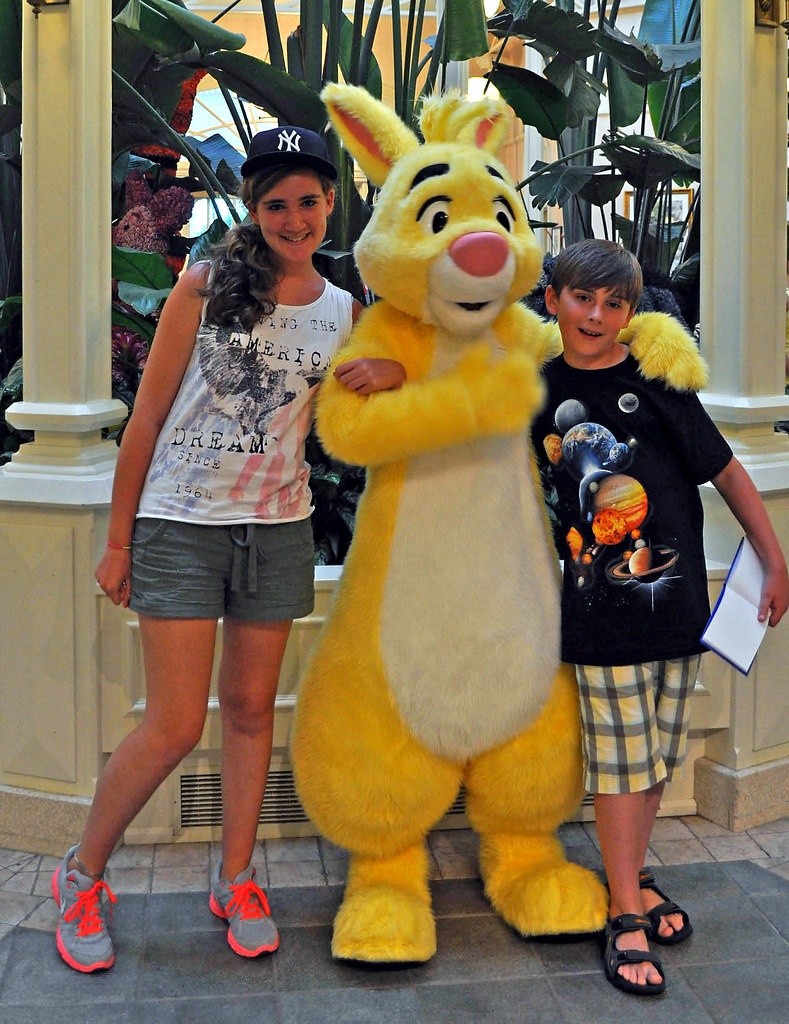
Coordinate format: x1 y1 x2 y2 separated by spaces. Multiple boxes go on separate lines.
290 81 710 971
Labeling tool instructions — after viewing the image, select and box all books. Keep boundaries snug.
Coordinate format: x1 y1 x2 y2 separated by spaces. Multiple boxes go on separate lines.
701 536 772 675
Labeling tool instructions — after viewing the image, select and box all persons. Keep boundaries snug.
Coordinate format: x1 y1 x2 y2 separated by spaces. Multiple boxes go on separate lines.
52 128 406 973
530 239 789 996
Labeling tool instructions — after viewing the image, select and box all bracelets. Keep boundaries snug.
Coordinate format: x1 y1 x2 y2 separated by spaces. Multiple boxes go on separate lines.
107 541 132 550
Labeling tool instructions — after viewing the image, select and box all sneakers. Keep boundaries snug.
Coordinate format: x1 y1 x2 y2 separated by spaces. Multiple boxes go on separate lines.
207 859 280 958
50 846 118 973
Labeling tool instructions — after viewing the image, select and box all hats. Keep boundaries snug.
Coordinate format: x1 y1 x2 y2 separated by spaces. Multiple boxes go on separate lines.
239 126 338 181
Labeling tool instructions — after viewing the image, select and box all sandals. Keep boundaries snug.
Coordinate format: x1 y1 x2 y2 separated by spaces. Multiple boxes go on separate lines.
603 865 693 946
598 900 666 996
